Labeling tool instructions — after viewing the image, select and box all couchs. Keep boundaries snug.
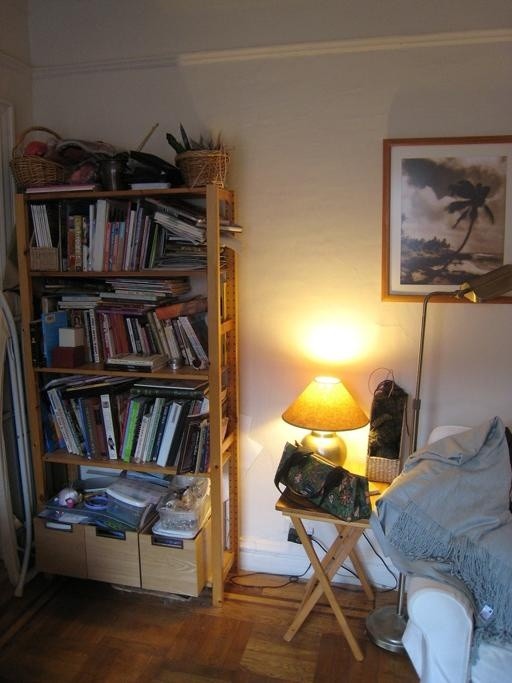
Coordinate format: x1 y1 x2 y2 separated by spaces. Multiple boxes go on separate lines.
405 426 512 683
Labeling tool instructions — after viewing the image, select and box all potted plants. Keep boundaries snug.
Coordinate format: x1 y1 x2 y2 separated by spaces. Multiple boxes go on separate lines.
166 124 231 187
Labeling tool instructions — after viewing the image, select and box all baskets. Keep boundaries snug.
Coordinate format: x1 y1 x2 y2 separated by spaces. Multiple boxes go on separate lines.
28 230 60 272
174 148 231 188
6 125 63 194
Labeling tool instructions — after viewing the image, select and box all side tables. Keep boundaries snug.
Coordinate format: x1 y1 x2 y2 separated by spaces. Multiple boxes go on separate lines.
275 481 391 660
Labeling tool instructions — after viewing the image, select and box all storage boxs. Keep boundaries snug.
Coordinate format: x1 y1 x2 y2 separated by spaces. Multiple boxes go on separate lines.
106 479 155 526
156 483 212 530
139 516 211 597
33 514 87 578
84 521 140 589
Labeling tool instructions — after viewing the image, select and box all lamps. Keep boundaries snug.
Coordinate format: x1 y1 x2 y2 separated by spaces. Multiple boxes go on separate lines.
282 378 372 466
364 265 512 655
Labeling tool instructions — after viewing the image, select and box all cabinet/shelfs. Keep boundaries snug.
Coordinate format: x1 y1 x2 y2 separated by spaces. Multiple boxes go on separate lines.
13 187 241 605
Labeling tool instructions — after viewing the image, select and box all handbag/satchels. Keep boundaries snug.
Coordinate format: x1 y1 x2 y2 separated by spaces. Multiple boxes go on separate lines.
273 441 373 521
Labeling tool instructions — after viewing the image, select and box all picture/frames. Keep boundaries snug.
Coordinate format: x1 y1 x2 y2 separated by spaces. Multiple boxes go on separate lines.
381 135 512 306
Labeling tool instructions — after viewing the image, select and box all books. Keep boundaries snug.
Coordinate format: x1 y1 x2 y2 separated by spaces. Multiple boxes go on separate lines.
40 374 228 473
25 184 99 194
42 278 222 373
30 197 243 272
